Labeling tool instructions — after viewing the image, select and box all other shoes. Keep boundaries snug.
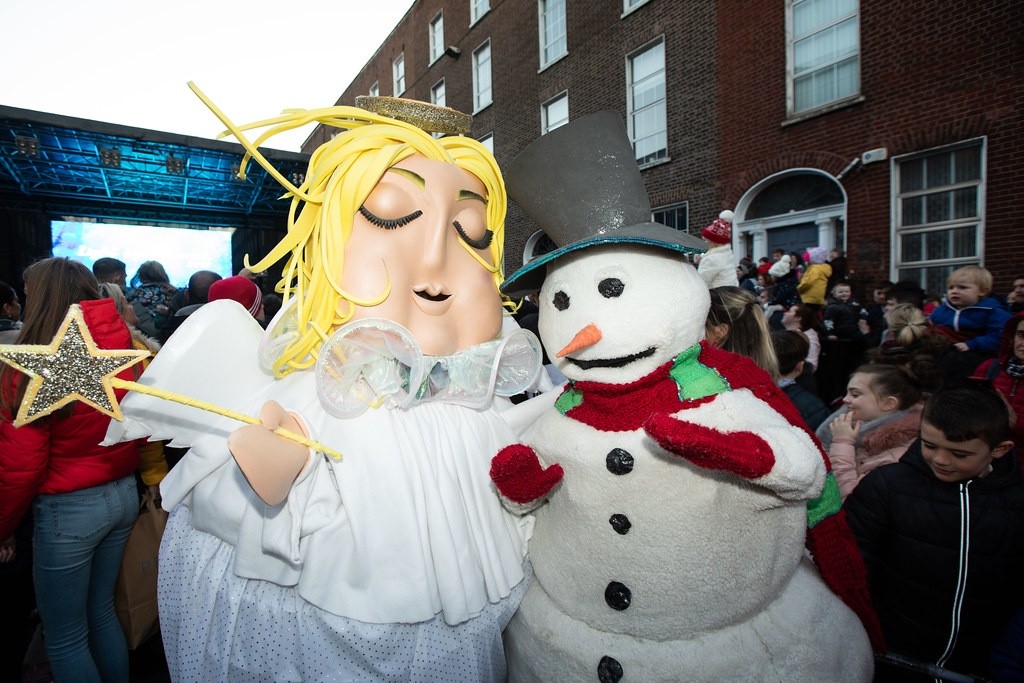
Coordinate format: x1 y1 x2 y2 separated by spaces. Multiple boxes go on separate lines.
25 663 54 683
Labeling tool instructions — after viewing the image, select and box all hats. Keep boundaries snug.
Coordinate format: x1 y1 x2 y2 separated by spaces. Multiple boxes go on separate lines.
701 209 735 245
209 276 262 319
768 254 791 276
806 246 828 264
790 252 802 264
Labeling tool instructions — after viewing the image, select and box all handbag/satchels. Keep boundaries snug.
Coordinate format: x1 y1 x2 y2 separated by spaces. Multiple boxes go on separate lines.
112 491 169 652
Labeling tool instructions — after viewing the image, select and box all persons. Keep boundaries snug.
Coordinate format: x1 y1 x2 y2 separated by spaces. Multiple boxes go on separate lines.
1 257 282 683
499 207 1024 683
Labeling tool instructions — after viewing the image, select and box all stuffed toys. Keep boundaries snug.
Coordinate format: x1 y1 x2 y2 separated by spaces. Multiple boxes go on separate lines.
490 110 888 682
